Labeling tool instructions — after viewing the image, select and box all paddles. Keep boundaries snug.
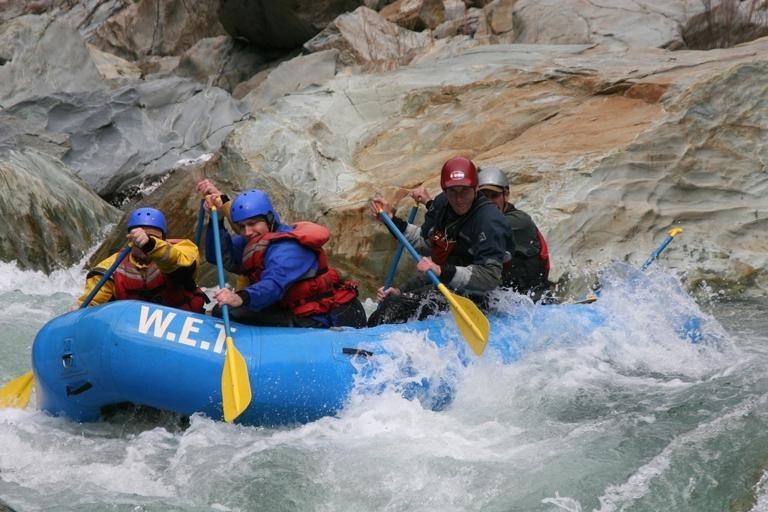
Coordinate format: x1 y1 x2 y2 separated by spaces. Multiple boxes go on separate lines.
0 239 134 411
210 195 252 421
377 208 489 356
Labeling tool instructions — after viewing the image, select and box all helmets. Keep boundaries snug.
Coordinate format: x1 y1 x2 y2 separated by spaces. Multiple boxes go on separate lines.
441 156 510 190
231 189 280 228
127 207 168 238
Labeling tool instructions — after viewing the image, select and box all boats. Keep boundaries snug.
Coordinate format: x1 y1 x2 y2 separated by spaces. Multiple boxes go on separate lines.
31 279 703 424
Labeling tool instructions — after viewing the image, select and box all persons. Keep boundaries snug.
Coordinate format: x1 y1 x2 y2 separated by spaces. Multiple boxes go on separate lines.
75 208 209 314
366 156 518 329
197 178 367 331
407 165 550 307
